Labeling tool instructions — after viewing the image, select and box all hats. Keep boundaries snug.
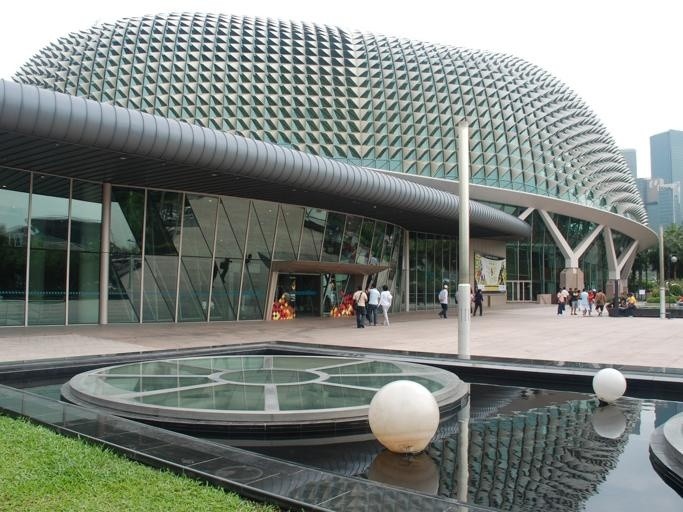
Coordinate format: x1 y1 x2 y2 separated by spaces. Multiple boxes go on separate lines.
444 285 448 288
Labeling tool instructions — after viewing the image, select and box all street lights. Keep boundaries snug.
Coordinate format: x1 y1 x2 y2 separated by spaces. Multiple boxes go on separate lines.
671 256 678 279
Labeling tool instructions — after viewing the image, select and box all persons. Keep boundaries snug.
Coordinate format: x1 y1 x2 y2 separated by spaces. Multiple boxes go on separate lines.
352 285 368 328
556 286 606 316
366 284 381 325
676 295 683 302
472 289 484 316
620 291 636 308
377 285 393 325
470 288 475 311
437 284 450 319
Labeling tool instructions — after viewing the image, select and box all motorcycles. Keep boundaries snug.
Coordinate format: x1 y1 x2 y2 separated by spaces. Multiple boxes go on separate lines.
621 298 626 307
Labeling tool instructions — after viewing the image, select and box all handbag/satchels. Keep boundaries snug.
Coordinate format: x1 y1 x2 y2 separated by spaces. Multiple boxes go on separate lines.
389 295 393 304
597 300 600 303
353 302 357 310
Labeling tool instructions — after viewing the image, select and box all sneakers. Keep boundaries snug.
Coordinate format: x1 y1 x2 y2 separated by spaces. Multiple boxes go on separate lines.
357 324 364 328
439 312 447 318
571 311 602 317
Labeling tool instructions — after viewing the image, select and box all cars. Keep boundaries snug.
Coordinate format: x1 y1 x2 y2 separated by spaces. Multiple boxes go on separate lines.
304 209 326 232
